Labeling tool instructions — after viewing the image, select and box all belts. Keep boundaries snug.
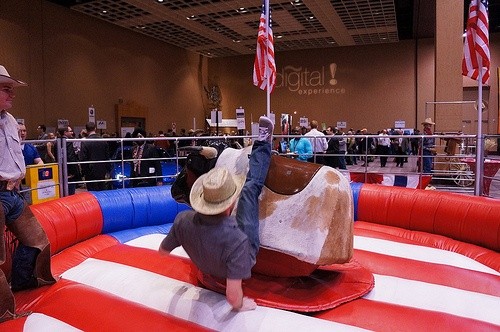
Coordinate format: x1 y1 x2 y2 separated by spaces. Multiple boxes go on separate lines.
0 179 21 192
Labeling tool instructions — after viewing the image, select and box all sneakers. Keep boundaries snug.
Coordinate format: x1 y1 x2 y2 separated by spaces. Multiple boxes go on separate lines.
257 116 274 142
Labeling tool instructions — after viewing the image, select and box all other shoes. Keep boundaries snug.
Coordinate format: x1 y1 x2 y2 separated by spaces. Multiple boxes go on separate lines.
11 276 38 292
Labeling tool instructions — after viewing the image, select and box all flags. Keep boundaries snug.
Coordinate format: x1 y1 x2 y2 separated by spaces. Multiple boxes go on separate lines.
462 0 490 87
252 1 277 94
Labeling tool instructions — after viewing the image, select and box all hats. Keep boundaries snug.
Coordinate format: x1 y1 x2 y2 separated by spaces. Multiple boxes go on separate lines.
189 167 247 216
421 118 436 125
0 65 28 87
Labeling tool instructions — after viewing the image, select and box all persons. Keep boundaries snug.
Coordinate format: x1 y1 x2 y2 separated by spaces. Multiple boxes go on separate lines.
157 116 275 311
0 64 57 322
416 118 437 190
289 120 421 170
33 122 252 196
18 122 44 185
